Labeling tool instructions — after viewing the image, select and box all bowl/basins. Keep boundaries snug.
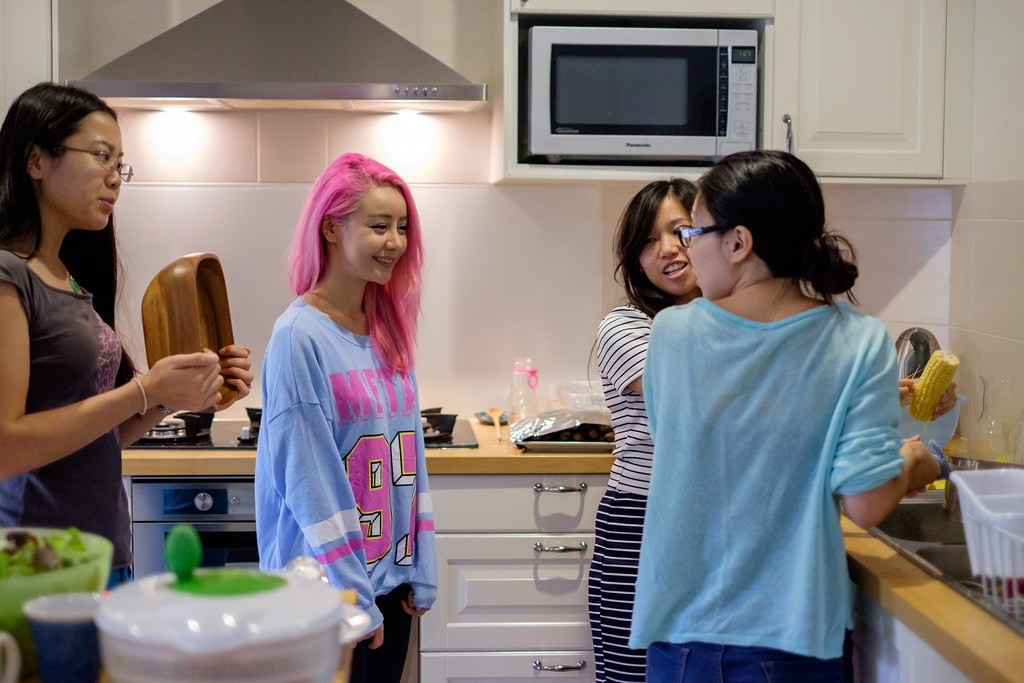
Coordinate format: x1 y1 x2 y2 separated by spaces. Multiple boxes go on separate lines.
0 526 114 675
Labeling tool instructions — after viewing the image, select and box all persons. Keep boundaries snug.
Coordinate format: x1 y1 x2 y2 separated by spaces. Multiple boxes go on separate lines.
627 148 939 683
254 152 439 683
586 176 958 683
0 83 254 683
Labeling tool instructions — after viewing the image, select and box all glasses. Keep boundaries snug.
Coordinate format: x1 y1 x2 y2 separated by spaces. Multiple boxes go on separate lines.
677 223 725 247
47 146 135 182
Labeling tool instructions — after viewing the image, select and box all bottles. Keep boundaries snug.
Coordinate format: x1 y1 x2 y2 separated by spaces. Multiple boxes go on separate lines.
508 357 541 423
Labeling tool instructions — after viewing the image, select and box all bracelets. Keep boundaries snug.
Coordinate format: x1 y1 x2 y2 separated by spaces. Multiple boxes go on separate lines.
131 378 147 415
157 404 175 415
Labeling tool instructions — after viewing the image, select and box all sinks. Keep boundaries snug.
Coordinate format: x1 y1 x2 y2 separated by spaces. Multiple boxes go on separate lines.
836 488 968 547
896 544 1024 639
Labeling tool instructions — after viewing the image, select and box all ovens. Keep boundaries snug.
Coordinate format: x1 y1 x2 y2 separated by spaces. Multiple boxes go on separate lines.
120 476 260 582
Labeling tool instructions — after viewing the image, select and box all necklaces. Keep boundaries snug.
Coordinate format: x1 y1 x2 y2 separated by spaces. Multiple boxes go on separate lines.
58 258 80 292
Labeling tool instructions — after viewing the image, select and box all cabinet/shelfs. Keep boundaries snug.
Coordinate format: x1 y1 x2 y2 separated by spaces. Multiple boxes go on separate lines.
417 472 611 683
762 0 946 185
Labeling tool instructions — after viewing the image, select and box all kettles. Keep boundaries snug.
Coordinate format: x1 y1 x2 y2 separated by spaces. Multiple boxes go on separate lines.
895 327 941 379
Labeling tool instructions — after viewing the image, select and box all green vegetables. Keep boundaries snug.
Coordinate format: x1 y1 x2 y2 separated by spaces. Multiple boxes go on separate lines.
0 526 98 579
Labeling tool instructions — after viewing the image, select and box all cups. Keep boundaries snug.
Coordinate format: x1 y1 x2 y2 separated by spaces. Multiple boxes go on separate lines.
23 592 100 683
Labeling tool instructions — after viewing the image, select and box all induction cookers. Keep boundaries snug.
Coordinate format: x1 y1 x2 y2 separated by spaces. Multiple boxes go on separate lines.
520 26 759 165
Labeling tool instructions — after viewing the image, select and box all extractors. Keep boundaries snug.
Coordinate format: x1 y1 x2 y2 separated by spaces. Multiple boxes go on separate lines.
49 0 488 115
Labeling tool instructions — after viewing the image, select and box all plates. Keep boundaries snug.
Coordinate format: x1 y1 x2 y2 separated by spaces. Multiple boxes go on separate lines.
475 413 508 424
515 441 615 452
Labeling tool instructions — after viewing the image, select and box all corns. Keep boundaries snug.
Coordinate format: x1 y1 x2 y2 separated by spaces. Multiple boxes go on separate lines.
909 350 960 423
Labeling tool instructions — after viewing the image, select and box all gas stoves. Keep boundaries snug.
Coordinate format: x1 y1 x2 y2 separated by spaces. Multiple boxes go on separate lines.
123 406 480 448
